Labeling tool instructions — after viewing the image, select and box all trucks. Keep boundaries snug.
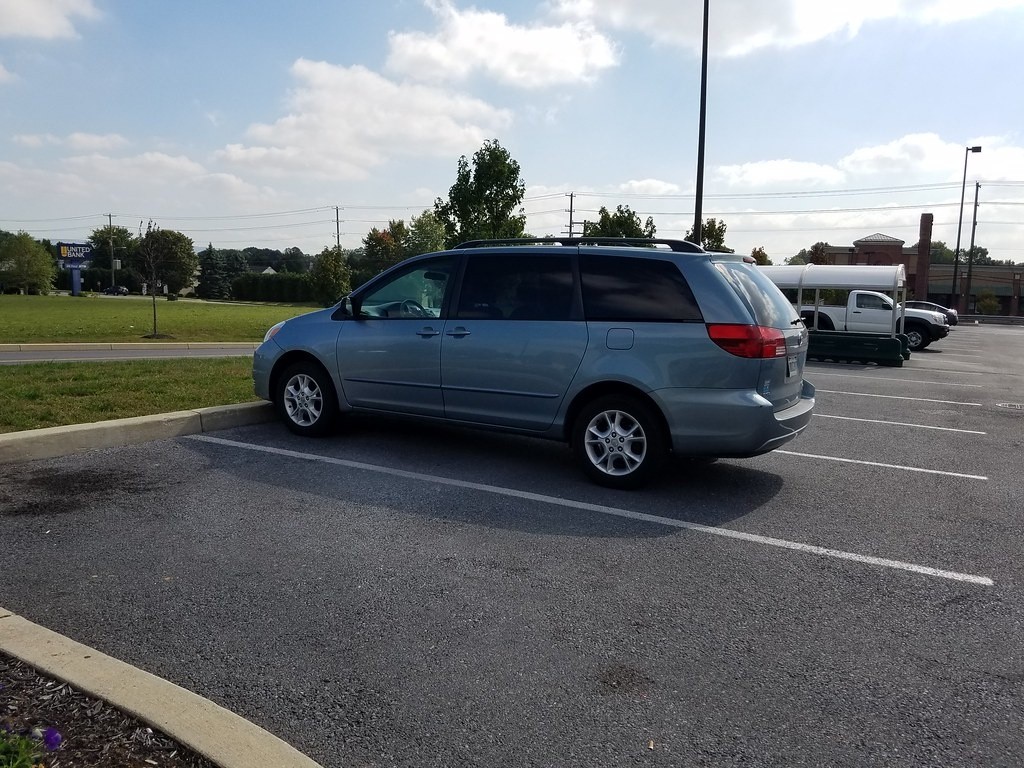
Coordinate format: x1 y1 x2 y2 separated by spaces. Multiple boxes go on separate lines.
790 289 949 352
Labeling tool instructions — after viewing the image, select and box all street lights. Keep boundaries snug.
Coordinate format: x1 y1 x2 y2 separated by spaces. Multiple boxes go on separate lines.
111 246 127 286
951 146 982 310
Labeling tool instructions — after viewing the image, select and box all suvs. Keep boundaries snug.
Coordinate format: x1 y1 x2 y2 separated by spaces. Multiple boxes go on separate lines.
251 235 817 489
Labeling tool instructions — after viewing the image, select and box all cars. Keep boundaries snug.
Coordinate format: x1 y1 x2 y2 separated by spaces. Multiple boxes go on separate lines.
897 299 959 327
103 285 129 295
